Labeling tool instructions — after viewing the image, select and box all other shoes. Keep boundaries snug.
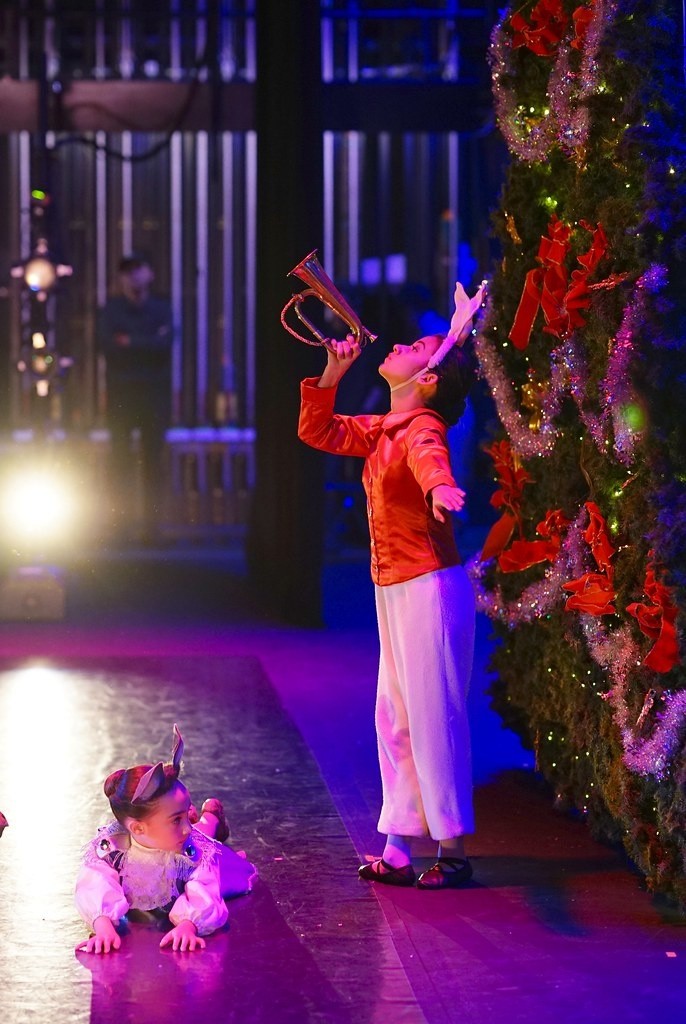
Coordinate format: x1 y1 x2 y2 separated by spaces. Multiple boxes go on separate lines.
414 856 475 889
201 798 232 845
360 862 414 888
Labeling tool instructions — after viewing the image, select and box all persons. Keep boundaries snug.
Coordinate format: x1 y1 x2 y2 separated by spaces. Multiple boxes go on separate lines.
99 251 178 544
74 722 256 953
298 335 477 887
